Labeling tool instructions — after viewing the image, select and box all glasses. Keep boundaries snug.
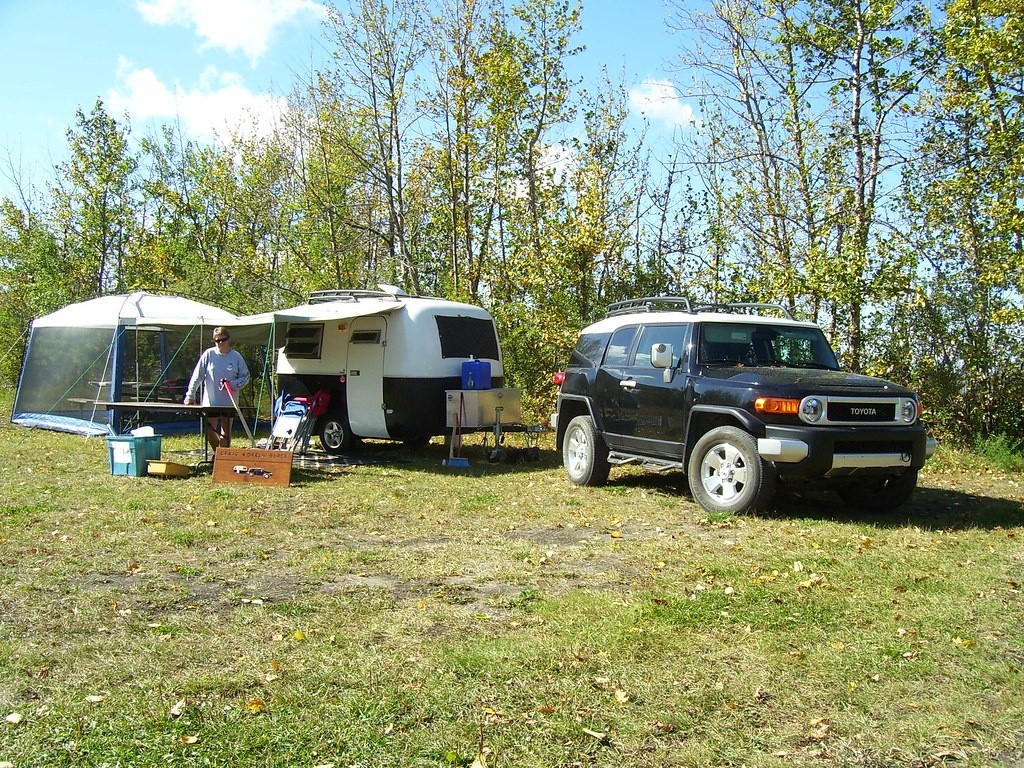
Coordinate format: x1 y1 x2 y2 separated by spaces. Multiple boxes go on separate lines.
215 336 228 343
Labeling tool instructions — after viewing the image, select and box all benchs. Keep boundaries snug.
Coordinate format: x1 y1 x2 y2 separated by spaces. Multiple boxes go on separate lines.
121 392 164 397
68 398 107 419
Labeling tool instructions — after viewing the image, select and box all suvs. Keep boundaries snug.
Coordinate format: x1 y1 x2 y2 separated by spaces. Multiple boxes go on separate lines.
555 297 936 516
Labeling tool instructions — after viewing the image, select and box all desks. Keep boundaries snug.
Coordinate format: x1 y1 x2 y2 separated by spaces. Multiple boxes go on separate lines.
162 386 188 420
88 381 161 401
93 401 261 478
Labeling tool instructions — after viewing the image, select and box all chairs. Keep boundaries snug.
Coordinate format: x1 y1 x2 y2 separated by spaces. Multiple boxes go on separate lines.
266 389 332 456
751 329 791 363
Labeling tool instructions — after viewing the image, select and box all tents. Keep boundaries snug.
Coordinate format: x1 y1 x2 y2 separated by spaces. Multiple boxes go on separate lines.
11 287 275 437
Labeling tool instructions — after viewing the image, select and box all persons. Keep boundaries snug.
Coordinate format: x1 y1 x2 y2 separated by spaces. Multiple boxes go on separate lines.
184 327 250 460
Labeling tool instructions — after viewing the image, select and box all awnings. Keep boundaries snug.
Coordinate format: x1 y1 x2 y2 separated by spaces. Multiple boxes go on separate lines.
136 302 405 454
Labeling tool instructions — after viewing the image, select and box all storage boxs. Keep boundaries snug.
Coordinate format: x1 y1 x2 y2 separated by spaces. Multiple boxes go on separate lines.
105 434 163 477
145 460 190 478
445 387 522 427
462 361 491 390
